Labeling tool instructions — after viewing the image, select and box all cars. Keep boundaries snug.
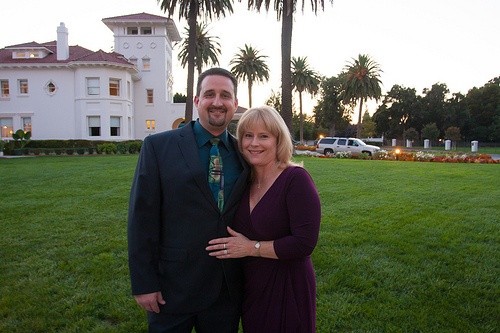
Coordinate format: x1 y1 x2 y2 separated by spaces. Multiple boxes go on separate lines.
315 136 381 156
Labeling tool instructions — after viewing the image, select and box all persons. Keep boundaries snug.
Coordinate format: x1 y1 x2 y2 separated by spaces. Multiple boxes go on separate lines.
127 68 250 333
206 107 321 333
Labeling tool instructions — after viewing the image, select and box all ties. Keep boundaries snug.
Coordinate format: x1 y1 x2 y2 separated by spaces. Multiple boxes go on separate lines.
207 138 224 213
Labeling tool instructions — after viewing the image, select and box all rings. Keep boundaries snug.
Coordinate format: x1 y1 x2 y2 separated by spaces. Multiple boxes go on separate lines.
224 243 226 248
224 250 228 254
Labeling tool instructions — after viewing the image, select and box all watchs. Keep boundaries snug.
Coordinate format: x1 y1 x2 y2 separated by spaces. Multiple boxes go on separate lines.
254 241 261 257
